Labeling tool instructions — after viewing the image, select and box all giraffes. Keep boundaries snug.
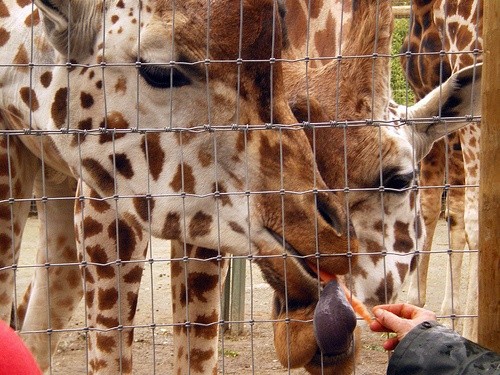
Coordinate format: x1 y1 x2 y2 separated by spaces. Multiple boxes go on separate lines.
270 0 485 374
0 1 357 372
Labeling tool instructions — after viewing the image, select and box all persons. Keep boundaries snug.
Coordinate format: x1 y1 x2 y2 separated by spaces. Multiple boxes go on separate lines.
369 302 500 375
